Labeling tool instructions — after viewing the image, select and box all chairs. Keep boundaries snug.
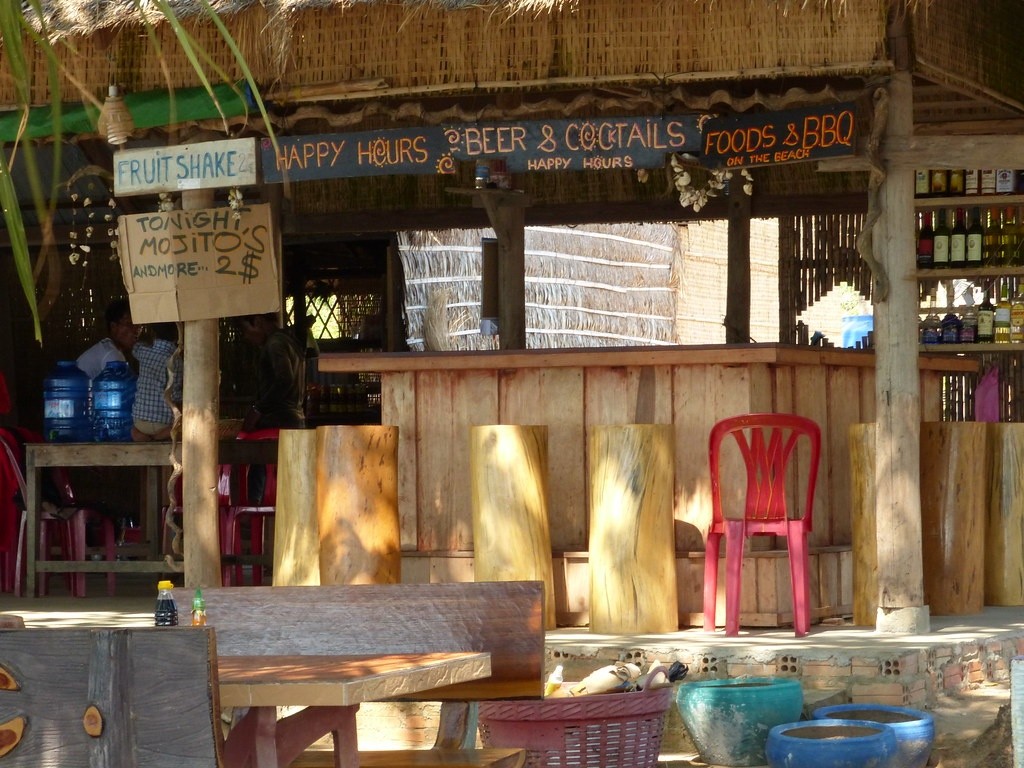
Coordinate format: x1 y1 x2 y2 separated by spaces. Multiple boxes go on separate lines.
1 375 313 598
704 415 822 637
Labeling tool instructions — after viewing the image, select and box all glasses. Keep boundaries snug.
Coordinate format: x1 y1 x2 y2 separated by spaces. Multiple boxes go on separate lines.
118 321 147 334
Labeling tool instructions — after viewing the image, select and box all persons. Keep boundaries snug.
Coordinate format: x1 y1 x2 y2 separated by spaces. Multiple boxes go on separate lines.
131 323 183 442
236 313 306 539
68 299 143 480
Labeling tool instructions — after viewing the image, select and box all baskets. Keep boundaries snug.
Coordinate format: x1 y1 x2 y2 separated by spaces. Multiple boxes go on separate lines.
477 665 674 768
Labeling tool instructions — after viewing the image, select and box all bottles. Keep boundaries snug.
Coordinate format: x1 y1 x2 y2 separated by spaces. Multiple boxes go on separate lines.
917 283 1024 344
319 383 369 412
92 361 133 442
914 169 1017 195
191 584 206 626
917 205 1024 267
42 360 92 441
154 580 179 626
474 155 512 190
542 666 564 696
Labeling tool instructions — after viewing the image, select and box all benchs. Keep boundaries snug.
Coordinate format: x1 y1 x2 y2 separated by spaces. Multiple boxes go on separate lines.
0 579 547 768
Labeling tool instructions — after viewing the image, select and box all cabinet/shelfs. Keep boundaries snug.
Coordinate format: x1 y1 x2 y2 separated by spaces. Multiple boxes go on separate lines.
912 121 1023 350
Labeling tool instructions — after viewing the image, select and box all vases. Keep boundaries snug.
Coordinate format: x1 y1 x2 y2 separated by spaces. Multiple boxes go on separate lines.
676 678 934 767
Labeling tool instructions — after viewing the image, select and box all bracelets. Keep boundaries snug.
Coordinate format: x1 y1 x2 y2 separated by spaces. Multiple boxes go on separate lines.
252 407 261 417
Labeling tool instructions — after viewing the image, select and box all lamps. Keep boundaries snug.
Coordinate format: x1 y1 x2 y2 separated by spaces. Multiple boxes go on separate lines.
98 85 134 146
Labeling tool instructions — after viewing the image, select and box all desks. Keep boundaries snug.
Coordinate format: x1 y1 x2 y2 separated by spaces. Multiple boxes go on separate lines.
213 647 492 768
27 441 184 597
